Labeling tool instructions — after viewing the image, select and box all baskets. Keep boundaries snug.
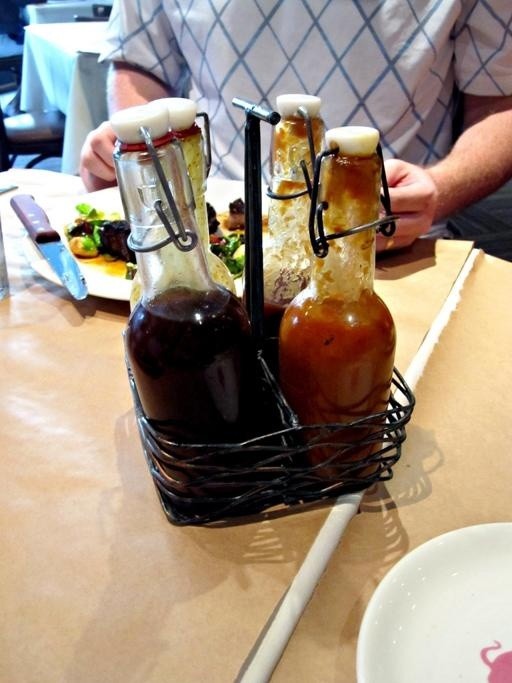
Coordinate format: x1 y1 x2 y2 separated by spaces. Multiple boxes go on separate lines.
124 297 415 524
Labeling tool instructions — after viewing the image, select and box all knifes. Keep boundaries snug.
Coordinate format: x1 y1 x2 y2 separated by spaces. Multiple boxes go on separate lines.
9 193 91 301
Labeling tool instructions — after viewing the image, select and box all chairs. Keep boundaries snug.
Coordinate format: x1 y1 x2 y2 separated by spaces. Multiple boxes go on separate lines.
0 106 64 173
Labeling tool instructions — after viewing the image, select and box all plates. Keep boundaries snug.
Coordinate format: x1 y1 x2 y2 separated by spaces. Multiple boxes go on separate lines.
356 517 511 681
19 179 278 305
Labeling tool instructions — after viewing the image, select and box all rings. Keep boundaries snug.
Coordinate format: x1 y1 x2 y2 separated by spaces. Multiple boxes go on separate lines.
384 235 394 248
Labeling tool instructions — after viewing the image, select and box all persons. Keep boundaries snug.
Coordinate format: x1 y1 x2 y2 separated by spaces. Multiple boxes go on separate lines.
79 0 511 252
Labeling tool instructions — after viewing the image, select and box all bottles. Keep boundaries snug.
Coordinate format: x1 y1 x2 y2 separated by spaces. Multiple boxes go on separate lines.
241 90 335 372
276 124 386 496
109 103 291 526
127 97 240 299
